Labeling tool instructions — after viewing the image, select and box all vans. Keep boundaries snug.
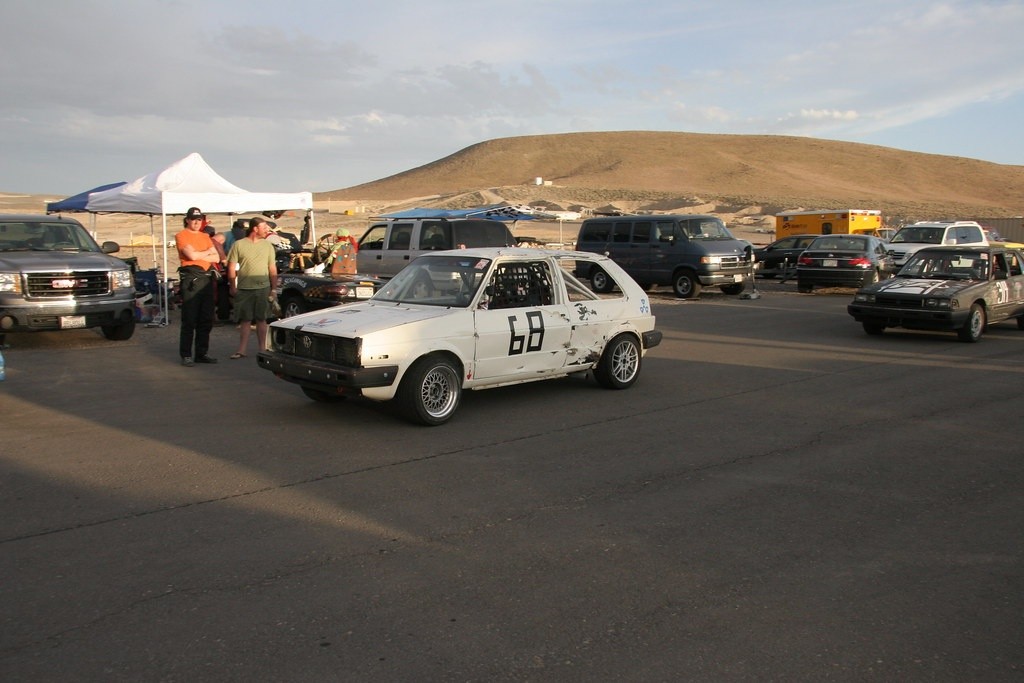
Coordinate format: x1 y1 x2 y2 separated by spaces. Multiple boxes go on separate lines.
573 214 751 298
886 220 994 279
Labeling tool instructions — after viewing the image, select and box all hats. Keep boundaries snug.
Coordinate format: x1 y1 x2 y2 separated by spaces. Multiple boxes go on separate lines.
187 207 203 220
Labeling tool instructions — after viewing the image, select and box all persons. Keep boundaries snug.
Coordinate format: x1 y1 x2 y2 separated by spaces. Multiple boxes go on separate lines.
228 217 278 359
875 254 891 281
324 227 358 274
175 207 221 367
182 215 250 322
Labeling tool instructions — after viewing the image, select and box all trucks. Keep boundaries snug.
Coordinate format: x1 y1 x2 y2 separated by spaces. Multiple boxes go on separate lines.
775 210 903 245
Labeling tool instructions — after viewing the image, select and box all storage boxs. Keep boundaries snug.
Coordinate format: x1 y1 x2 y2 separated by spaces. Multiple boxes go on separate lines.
136 304 161 323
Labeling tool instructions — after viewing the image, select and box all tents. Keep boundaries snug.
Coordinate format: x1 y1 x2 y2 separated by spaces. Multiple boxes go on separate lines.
48 181 159 267
369 205 580 265
86 152 317 326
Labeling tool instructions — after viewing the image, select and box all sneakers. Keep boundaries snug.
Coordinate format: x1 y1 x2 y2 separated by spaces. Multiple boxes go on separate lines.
182 356 196 366
194 354 218 363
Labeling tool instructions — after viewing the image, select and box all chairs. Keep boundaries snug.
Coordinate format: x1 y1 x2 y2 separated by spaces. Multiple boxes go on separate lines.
135 269 179 303
392 232 409 250
421 234 445 250
41 231 58 245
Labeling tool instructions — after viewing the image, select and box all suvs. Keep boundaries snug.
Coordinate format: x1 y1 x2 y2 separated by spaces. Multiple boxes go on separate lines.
355 218 519 287
212 230 388 324
983 228 1024 253
0 213 139 340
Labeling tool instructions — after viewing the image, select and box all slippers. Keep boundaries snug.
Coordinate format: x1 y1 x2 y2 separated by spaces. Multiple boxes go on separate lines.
231 352 247 358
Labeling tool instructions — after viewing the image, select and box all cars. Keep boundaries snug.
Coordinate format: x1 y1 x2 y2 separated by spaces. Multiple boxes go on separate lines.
751 235 821 278
847 245 1024 342
797 234 895 293
257 245 664 426
